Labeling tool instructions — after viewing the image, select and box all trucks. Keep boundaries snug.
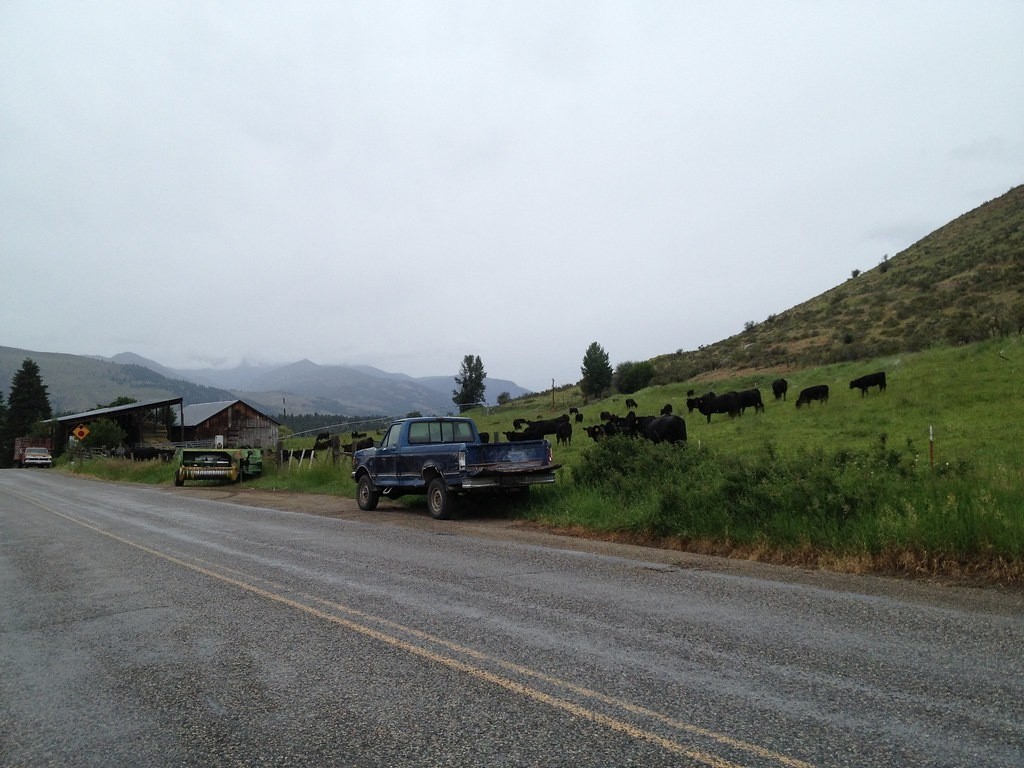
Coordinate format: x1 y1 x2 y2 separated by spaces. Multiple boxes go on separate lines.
13 436 54 469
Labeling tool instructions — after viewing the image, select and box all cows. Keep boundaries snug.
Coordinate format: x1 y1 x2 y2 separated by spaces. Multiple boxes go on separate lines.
772 377 788 402
735 388 765 416
795 384 829 410
281 388 717 465
849 371 887 398
711 391 751 420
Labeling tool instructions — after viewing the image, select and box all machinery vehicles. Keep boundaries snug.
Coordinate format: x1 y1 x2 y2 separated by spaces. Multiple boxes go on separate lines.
174 441 263 485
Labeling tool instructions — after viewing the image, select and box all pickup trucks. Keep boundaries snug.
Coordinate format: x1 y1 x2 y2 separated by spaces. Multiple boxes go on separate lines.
352 415 556 520
